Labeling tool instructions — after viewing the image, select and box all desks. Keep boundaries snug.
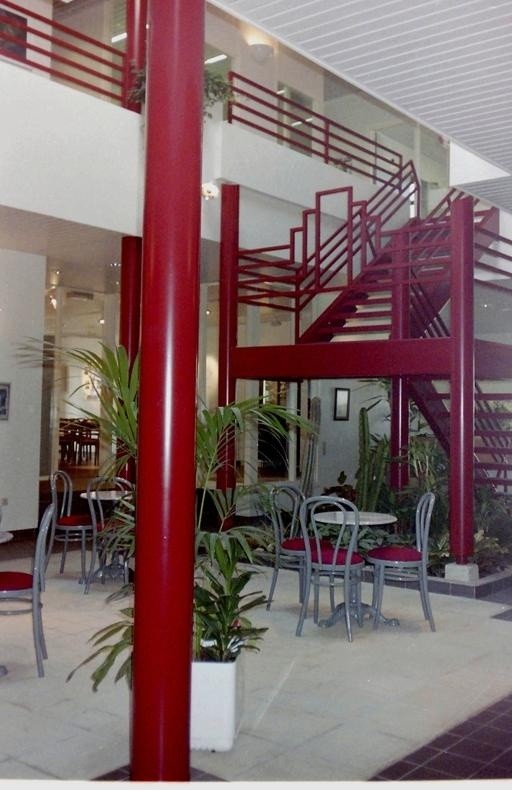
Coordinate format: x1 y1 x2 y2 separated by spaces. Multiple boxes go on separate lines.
80 490 135 585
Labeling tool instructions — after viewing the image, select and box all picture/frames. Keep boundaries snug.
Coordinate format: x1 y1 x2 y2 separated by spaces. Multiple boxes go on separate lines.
334 388 350 421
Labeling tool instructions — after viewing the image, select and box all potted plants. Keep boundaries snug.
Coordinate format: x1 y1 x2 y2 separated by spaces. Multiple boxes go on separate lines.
15 335 324 755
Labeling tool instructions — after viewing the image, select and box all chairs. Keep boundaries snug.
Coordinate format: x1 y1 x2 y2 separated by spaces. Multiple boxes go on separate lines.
84 476 135 595
44 469 106 585
0 502 57 678
266 482 436 642
59 419 99 466
